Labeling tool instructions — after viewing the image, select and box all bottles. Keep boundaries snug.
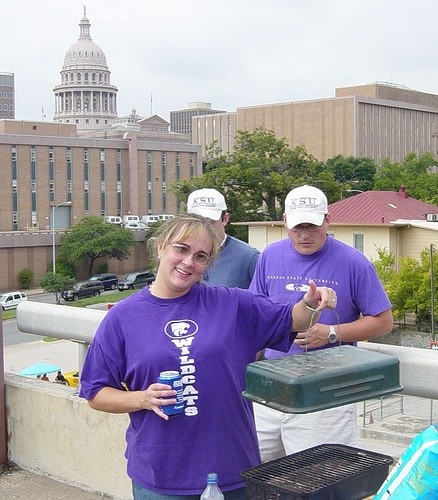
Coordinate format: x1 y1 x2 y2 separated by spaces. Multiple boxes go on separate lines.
200 473 224 500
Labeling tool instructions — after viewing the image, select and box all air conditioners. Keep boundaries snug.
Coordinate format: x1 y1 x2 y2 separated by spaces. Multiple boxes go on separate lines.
426 214 438 221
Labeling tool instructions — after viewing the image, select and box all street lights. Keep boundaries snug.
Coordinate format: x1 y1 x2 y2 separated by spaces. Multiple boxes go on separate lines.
340 180 359 201
53 201 73 274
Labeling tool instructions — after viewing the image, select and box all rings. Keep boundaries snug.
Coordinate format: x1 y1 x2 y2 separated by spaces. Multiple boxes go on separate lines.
332 300 335 303
303 339 305 344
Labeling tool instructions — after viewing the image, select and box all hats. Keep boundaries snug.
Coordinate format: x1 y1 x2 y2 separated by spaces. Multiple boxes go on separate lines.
285 185 328 229
187 188 227 221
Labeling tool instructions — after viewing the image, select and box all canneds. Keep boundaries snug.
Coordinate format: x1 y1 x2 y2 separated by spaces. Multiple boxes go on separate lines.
159 371 179 378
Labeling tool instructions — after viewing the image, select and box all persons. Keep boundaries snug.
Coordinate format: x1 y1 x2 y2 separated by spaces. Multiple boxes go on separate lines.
78 213 338 500
41 370 66 384
187 188 261 290
247 186 394 464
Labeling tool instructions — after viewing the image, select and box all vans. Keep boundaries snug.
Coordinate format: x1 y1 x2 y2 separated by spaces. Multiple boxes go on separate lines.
0 291 27 311
104 214 175 230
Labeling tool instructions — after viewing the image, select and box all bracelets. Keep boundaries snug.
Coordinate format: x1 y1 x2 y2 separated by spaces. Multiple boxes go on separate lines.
302 295 317 313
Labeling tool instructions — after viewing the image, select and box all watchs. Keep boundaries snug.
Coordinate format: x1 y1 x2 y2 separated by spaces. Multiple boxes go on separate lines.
328 325 338 344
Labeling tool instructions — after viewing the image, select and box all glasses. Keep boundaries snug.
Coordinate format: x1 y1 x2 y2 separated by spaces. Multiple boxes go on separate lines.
291 224 322 232
167 243 213 267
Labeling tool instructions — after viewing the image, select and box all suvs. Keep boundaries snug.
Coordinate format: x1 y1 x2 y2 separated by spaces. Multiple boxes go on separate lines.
60 279 105 301
85 273 118 291
117 271 155 292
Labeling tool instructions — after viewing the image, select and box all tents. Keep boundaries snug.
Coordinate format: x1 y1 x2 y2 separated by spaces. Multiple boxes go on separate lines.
20 363 62 380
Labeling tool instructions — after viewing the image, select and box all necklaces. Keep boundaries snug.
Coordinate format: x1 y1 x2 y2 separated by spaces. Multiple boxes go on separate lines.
149 281 154 291
